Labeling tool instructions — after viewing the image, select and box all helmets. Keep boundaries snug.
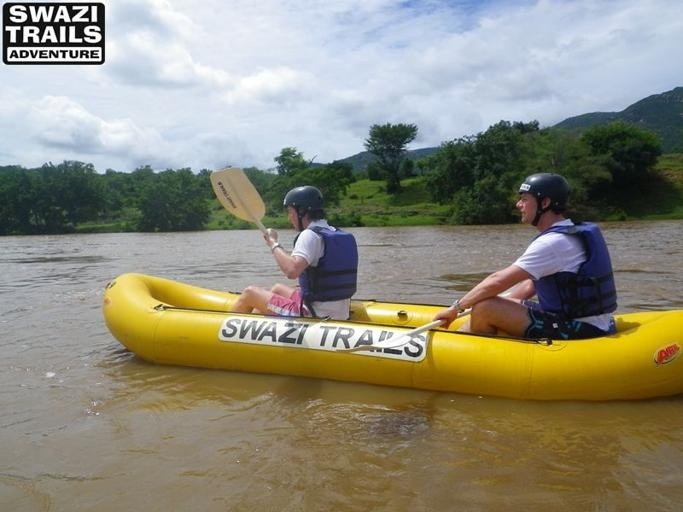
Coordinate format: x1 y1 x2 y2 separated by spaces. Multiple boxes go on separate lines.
283 185 325 219
519 171 569 215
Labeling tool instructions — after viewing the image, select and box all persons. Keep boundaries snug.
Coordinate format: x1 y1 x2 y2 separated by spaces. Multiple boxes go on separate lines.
432 172 617 342
229 186 358 321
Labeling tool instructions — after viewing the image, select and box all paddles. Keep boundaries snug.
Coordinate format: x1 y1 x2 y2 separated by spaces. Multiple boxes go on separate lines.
335 294 511 353
210 168 315 318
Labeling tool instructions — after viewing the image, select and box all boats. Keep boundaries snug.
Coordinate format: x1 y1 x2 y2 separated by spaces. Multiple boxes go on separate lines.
101 273 683 403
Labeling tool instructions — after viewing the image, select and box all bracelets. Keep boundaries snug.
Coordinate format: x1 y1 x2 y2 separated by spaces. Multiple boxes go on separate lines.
453 299 465 313
271 242 283 253
